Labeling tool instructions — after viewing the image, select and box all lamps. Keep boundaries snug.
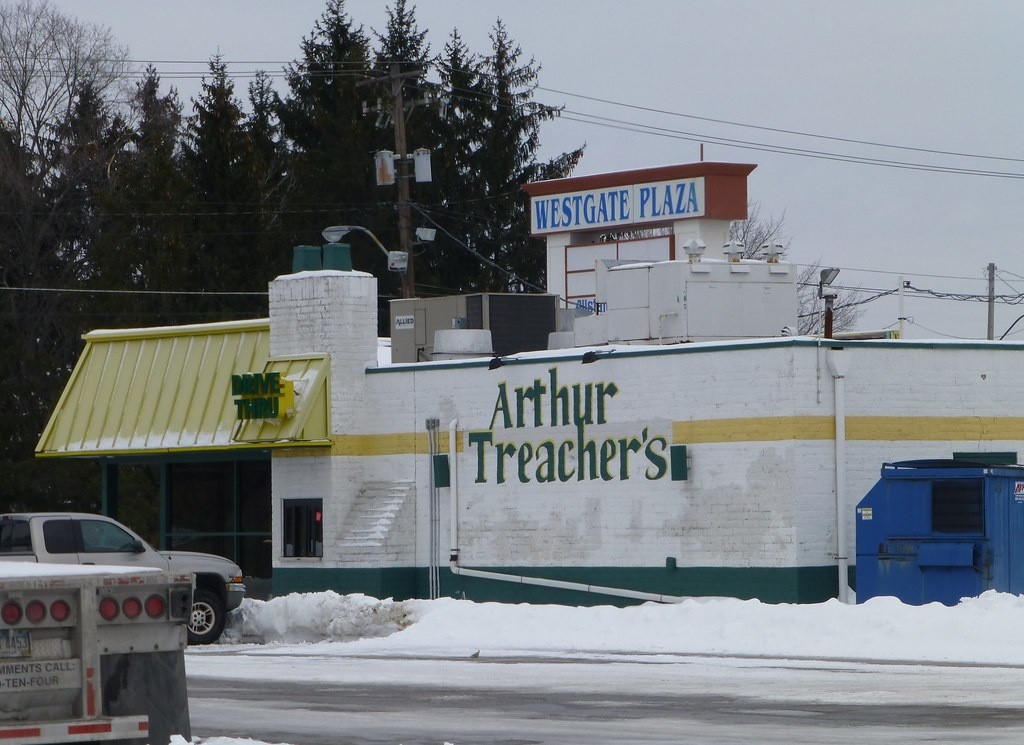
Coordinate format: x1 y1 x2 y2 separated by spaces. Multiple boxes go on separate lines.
432 454 450 488
581 350 613 365
820 267 840 285
487 357 516 371
670 445 691 481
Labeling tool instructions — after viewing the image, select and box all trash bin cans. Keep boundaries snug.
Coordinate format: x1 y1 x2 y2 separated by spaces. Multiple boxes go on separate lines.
855 458 1024 606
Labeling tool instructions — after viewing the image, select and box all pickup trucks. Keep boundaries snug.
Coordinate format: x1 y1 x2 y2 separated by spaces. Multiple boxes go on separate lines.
0 512 245 645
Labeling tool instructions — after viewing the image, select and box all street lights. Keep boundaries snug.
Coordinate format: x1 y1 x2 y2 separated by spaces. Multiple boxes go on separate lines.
322 225 418 299
817 267 841 340
